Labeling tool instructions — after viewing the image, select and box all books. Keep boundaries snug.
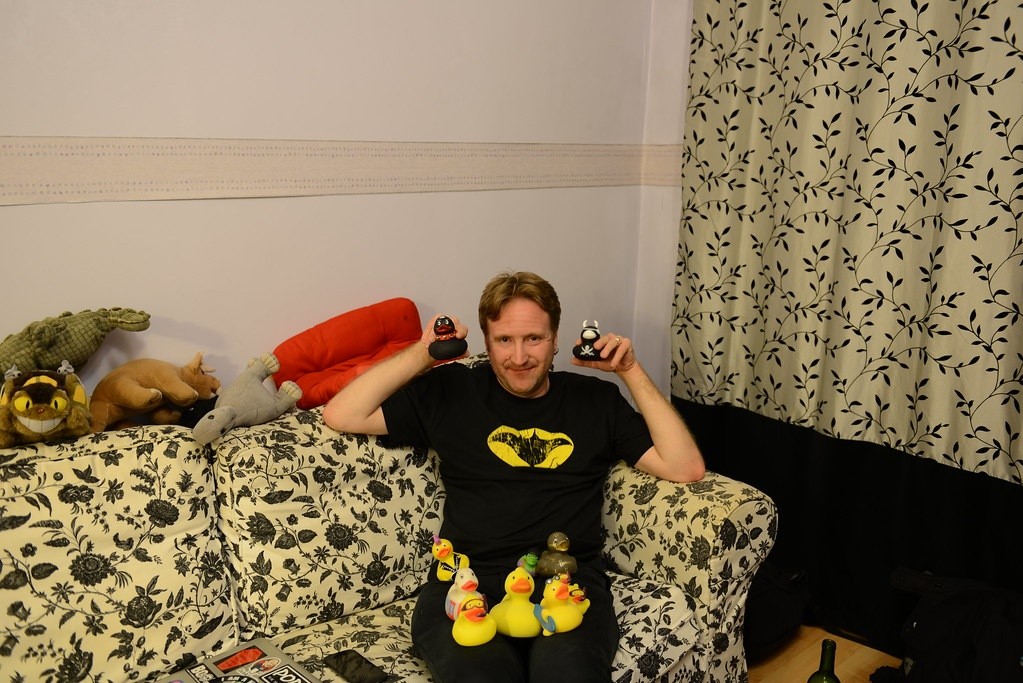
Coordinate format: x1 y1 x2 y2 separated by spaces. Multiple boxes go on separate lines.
155 637 322 683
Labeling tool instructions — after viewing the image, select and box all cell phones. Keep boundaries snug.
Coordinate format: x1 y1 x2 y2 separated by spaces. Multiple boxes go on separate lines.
321 649 388 683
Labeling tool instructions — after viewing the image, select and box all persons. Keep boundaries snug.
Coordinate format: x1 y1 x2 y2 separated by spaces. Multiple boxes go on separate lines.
251 658 279 674
323 271 707 683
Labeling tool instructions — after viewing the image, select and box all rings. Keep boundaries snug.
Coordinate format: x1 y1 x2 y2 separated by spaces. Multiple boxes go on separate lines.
615 337 620 346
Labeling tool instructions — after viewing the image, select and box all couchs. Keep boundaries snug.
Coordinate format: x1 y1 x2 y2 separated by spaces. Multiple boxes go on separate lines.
0 352 778 683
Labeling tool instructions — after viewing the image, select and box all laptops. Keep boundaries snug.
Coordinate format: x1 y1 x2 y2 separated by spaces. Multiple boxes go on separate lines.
151 638 321 683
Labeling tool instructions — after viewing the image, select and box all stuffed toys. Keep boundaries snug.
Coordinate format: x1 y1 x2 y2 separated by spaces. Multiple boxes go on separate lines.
488 567 541 637
432 536 470 581
572 319 604 361
538 532 578 574
452 594 496 646
0 359 93 448
0 307 150 390
516 552 538 574
445 568 488 621
89 351 222 433
533 570 591 636
428 314 467 360
193 350 302 445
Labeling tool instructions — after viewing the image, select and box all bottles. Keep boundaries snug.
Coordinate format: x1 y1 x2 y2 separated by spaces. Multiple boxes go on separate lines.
807 639 841 683
903 616 920 683
